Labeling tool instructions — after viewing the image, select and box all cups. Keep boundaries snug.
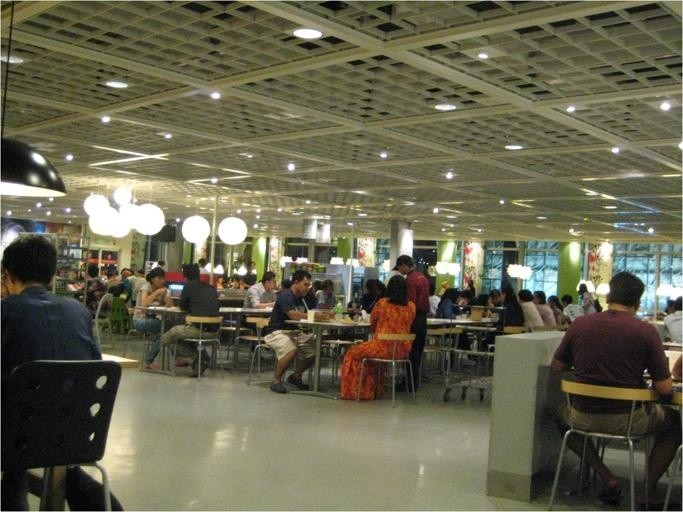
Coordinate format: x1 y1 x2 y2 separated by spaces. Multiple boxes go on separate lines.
455 315 461 321
461 314 466 319
307 310 314 322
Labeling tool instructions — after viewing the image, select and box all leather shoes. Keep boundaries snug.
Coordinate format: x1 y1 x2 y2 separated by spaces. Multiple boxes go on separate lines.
271 374 309 393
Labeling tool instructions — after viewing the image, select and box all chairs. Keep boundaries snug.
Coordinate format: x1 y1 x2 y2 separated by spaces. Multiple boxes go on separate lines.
41 292 682 408
0 359 124 512
547 374 652 509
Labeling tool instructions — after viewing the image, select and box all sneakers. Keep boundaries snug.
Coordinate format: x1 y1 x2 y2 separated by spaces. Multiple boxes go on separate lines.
191 361 205 376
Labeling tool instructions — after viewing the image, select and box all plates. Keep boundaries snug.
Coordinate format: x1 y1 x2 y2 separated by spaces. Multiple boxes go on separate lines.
340 320 358 325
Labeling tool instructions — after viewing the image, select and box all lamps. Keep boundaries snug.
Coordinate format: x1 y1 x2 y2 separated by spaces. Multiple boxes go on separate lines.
83 181 166 237
218 192 247 246
183 189 211 245
3 51 68 200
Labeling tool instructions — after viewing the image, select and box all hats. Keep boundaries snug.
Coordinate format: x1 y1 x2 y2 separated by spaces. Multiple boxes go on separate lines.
393 255 411 270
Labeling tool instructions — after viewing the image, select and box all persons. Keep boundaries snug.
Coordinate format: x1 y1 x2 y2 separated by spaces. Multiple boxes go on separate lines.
0 230 127 511
57 250 682 403
545 271 681 510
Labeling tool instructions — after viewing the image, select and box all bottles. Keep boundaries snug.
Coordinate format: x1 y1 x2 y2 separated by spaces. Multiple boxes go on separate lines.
335 303 342 322
